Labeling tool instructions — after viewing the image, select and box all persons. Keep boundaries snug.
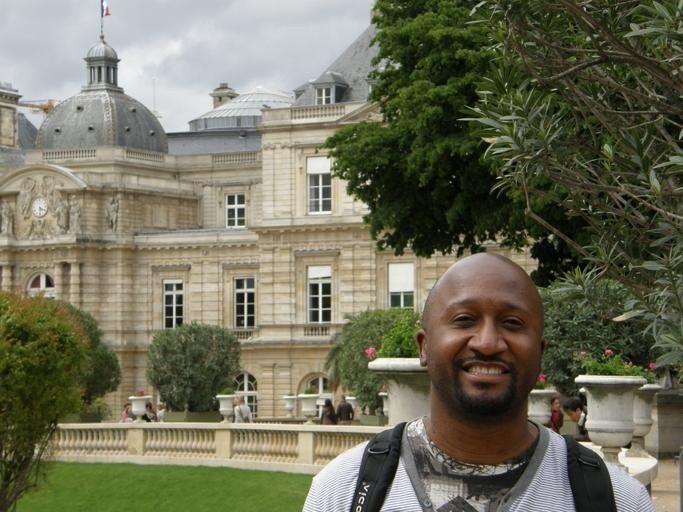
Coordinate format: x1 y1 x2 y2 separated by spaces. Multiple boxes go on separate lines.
156 403 165 422
230 392 253 423
299 252 655 511
141 402 157 422
120 403 132 422
319 398 337 425
562 396 586 435
335 394 354 425
543 395 564 435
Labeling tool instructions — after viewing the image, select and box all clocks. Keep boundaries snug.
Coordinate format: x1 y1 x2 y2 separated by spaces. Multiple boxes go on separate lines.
31 198 49 217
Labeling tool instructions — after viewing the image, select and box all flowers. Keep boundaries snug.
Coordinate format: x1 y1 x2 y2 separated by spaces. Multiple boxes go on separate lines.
134 387 145 397
364 314 420 360
575 347 659 384
533 374 554 388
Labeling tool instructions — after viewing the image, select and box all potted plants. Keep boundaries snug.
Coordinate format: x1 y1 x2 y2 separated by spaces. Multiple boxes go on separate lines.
215 387 238 423
282 388 320 424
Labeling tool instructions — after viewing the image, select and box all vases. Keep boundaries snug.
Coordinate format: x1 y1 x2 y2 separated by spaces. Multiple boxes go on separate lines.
574 373 662 472
127 396 153 423
527 388 560 427
367 356 431 428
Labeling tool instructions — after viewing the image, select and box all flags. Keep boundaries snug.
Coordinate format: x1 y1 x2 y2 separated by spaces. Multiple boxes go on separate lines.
98 0 111 19
